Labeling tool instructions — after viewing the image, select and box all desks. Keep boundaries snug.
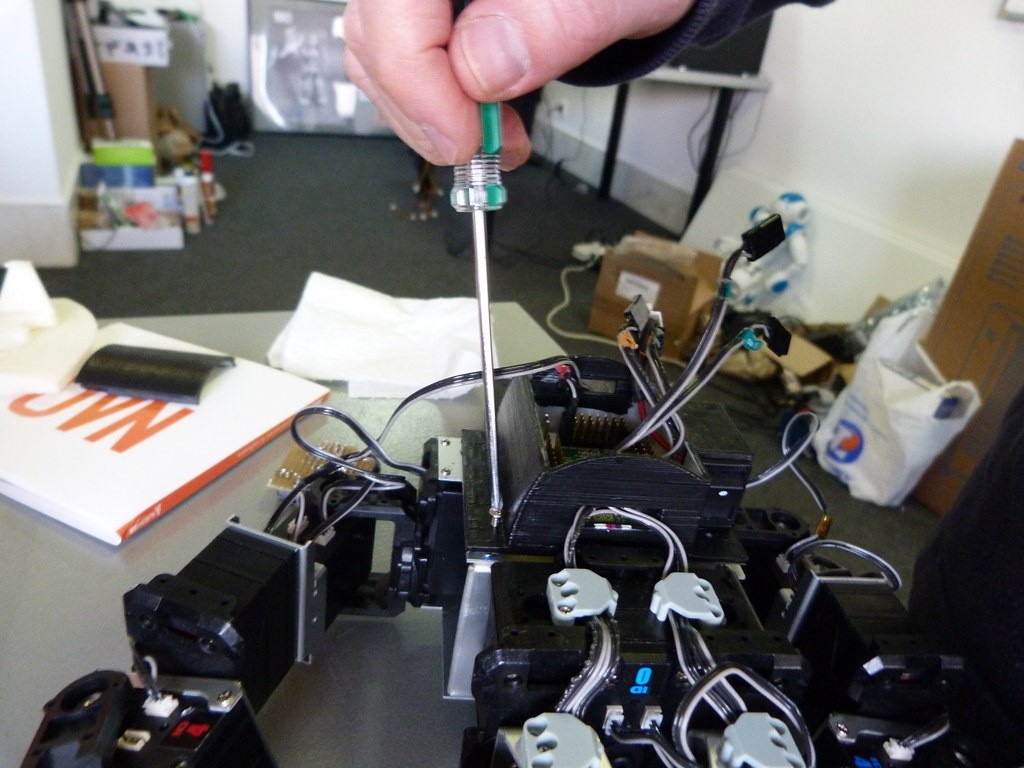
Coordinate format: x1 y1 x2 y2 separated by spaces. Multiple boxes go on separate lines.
487 68 769 257
0 300 571 768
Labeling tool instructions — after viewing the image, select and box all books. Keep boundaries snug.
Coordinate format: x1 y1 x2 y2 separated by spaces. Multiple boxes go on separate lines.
0 320 329 545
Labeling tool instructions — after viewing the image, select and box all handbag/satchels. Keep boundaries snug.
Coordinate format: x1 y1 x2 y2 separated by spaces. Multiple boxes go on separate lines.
811 304 982 510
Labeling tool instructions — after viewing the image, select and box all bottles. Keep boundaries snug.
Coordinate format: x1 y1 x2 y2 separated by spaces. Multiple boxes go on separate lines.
198 150 218 226
180 171 201 234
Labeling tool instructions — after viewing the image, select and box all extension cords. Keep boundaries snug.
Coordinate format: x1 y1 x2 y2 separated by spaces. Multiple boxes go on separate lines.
570 242 609 263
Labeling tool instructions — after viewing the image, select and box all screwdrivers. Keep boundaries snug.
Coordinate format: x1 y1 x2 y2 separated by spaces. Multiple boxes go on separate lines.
450 101 507 509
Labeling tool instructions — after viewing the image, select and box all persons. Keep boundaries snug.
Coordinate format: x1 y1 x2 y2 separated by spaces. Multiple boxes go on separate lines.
340 0 1023 768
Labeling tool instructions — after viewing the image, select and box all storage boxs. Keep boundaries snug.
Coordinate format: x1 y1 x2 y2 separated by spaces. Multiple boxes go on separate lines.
588 230 720 352
75 20 199 250
762 295 898 390
